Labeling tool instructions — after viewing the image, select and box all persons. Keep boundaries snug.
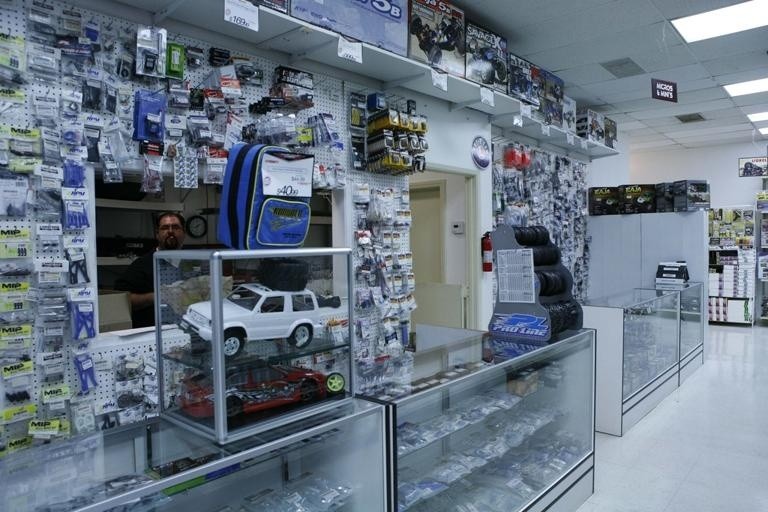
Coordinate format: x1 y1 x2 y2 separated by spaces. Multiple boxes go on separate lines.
114 212 195 328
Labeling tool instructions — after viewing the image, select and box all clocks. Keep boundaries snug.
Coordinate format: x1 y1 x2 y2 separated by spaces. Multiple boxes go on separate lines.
185 215 208 238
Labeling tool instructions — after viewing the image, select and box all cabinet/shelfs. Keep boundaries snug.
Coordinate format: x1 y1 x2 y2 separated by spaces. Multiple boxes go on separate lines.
578 288 680 438
635 281 705 389
0 391 391 512
150 242 355 448
349 324 597 512
708 198 767 329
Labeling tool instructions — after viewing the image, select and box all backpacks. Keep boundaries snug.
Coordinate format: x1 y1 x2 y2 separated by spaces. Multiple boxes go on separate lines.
216 142 315 250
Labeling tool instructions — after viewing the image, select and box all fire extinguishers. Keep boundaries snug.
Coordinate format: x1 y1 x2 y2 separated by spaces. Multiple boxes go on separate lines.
481 232 493 271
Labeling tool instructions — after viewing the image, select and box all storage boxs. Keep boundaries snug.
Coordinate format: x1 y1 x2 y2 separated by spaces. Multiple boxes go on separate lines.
409 0 466 79
506 46 577 136
576 107 604 145
587 179 714 216
255 0 290 16
604 116 618 150
291 0 409 60
466 20 507 95
